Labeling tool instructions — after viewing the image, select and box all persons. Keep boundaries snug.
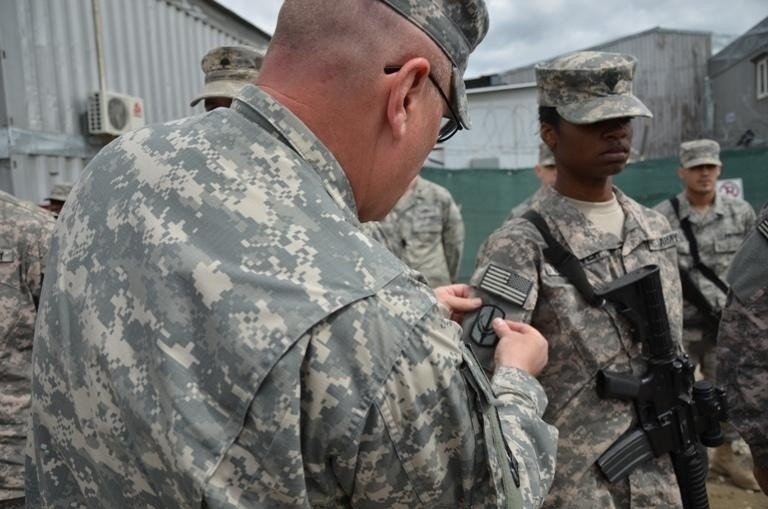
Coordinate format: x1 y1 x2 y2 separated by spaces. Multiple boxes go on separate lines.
26 0 555 509
188 34 269 114
459 48 694 508
2 188 52 507
525 136 559 188
710 201 766 502
647 138 761 495
40 180 77 215
362 174 469 301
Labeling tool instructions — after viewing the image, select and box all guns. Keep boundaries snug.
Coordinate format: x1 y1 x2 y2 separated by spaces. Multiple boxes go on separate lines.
592 265 731 507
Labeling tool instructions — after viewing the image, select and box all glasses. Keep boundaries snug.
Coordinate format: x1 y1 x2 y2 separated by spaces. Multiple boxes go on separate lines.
386 66 460 143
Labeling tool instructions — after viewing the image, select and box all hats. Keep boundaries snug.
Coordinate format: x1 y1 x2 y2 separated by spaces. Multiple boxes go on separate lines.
381 0 490 129
190 45 261 107
679 138 723 168
533 50 654 124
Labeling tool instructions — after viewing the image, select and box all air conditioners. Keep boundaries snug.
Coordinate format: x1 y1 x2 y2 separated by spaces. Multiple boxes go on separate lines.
86 91 145 136
